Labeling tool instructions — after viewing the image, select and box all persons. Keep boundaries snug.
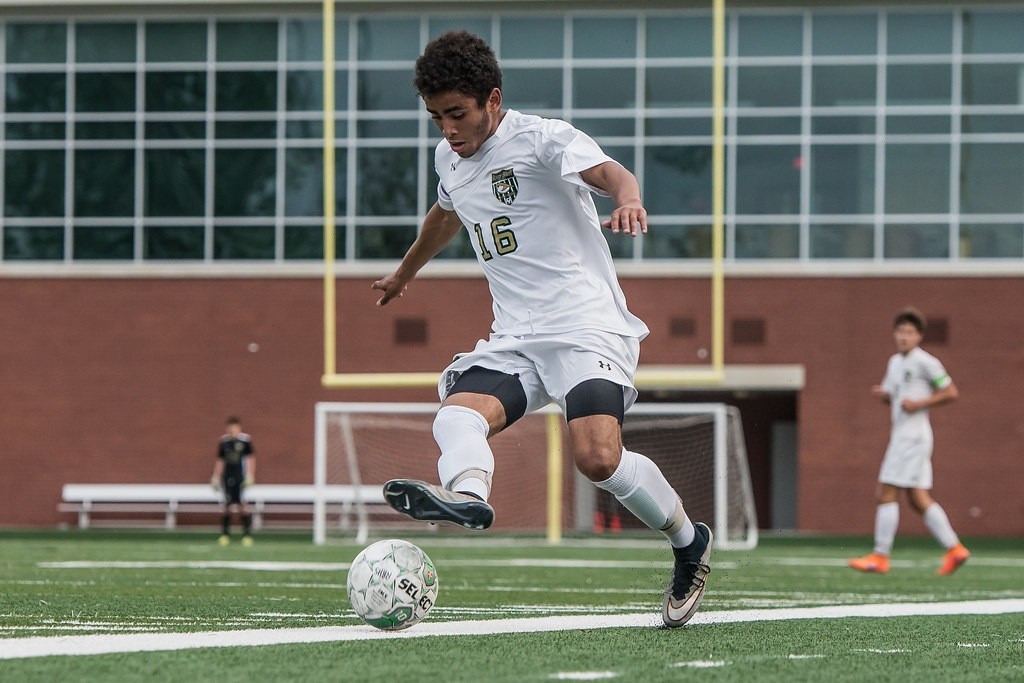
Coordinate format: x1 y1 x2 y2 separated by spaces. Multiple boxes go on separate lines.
370 28 714 629
847 308 972 576
211 417 257 546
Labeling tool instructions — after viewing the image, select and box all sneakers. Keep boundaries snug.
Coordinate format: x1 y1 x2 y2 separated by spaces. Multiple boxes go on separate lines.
661 521 714 627
848 552 890 573
383 478 494 532
937 545 970 576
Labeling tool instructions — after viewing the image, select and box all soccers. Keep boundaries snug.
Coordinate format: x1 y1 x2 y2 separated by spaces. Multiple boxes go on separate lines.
347 539 439 630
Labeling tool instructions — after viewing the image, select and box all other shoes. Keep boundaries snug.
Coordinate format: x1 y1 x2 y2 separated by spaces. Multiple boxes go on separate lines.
242 536 254 547
218 535 231 545
